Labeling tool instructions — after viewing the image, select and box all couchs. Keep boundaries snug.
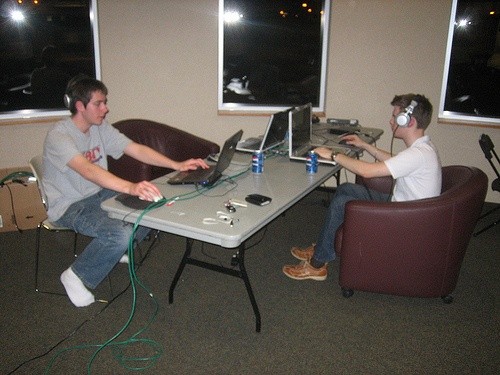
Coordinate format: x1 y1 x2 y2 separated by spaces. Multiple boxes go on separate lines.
333 165 489 306
107 119 220 183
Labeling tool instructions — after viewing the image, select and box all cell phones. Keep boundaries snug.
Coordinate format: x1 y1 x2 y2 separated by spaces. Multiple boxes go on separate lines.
245 194 272 205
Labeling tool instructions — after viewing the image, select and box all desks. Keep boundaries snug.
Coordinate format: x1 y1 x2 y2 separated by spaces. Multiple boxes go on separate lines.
100 117 384 336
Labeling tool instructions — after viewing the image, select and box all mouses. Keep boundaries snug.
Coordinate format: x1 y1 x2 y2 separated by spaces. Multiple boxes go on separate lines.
138 192 163 202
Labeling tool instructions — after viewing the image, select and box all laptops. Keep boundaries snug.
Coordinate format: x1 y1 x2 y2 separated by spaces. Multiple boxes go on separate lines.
287 103 352 166
234 107 295 153
166 130 244 185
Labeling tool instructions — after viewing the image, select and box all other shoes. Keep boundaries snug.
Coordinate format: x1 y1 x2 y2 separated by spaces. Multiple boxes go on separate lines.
282 260 328 281
290 245 329 265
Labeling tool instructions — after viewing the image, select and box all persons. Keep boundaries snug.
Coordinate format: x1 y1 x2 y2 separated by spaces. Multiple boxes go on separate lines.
283 94 442 280
44 77 209 308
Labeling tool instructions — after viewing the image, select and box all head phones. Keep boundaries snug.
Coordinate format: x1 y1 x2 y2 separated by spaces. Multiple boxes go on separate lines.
64 74 87 108
395 93 422 128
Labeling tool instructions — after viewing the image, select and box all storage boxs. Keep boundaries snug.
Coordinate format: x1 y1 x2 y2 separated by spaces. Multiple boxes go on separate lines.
0 166 49 233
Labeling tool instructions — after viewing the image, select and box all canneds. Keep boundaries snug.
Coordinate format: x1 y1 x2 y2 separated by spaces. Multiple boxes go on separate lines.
251 150 264 175
305 151 318 174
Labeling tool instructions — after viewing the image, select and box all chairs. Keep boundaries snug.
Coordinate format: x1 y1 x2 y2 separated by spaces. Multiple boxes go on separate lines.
29 154 117 304
478 133 500 192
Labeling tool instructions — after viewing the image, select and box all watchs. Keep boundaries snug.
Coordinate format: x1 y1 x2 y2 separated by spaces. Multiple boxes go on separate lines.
331 151 339 161
